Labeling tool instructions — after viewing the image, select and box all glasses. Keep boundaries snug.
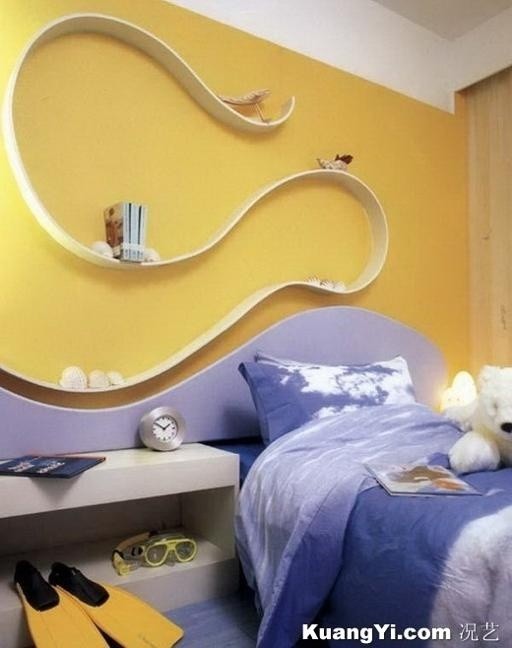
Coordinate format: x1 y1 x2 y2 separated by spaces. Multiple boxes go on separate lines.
144 539 197 566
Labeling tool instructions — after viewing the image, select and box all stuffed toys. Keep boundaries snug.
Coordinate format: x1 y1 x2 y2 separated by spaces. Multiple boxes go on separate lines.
446 363 512 473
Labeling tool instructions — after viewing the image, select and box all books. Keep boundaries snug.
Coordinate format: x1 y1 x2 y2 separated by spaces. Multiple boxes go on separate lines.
106 201 148 263
362 463 483 498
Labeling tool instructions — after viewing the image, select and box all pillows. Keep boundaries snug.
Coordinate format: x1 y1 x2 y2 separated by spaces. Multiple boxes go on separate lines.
254 349 277 361
236 349 427 445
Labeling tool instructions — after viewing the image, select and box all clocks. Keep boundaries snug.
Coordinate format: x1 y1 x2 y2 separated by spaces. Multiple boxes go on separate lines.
138 406 186 450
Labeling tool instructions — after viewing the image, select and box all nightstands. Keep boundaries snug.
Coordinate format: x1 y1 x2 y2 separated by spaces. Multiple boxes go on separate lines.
0 443 240 648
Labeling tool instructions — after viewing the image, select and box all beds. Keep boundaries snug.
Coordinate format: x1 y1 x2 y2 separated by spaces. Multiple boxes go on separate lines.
207 404 511 647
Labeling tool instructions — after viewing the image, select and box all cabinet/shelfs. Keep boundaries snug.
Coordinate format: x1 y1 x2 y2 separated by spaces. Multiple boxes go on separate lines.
1 14 387 397
